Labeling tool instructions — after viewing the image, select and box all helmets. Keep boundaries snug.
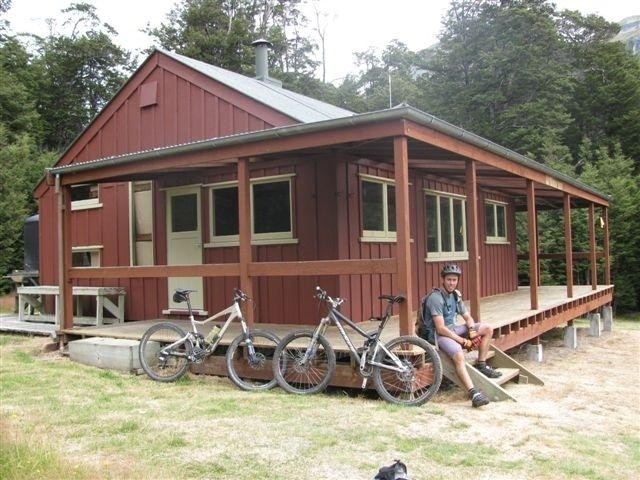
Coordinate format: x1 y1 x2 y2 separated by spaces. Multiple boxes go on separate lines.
441 264 461 276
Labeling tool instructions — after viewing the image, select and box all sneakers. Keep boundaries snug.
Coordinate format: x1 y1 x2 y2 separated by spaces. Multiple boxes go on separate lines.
475 362 502 378
469 390 489 407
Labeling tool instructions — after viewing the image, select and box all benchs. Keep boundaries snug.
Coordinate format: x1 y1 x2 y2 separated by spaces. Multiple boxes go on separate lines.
16 286 126 326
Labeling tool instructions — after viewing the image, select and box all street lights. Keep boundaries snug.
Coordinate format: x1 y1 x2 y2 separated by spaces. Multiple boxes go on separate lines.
387 67 400 106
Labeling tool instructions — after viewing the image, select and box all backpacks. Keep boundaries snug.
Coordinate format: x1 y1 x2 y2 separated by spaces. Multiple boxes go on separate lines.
415 287 458 340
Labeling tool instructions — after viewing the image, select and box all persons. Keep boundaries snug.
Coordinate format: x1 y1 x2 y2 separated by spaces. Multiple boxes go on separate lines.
422 263 503 409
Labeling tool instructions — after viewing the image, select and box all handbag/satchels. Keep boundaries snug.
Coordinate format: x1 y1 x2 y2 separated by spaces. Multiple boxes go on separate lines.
375 460 410 480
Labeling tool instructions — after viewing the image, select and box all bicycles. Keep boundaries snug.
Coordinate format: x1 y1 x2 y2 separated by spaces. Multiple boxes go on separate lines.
139 291 286 392
270 286 445 407
